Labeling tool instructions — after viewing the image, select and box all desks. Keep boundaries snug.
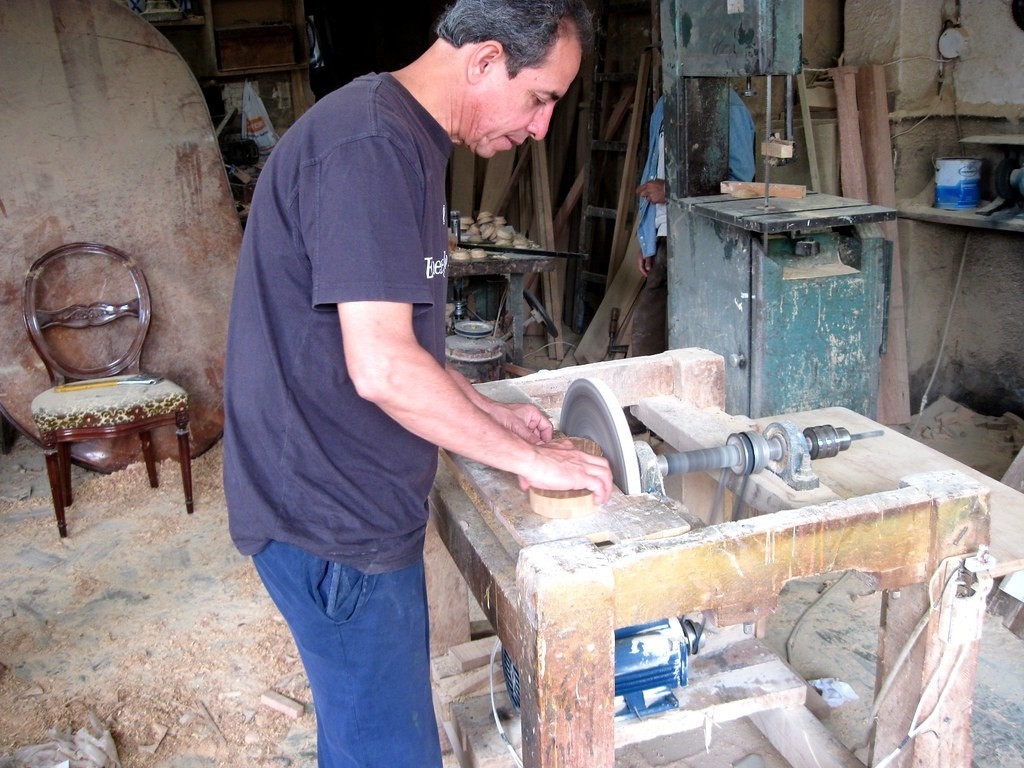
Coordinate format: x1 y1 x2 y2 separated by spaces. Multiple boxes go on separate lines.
449 235 557 365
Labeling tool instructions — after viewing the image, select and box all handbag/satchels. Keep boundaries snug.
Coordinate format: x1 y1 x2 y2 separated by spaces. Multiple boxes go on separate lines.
241 79 277 156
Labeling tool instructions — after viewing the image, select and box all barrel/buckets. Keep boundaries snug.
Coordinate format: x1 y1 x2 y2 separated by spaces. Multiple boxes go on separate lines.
931 149 981 212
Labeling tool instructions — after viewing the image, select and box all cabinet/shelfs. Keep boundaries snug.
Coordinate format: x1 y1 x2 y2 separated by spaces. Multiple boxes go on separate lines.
202 0 311 78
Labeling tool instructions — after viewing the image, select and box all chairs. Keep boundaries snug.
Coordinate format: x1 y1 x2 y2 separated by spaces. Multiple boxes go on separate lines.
22 242 193 538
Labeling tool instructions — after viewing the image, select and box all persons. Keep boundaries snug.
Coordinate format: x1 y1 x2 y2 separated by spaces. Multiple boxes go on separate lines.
623 77 756 434
224 0 613 768
